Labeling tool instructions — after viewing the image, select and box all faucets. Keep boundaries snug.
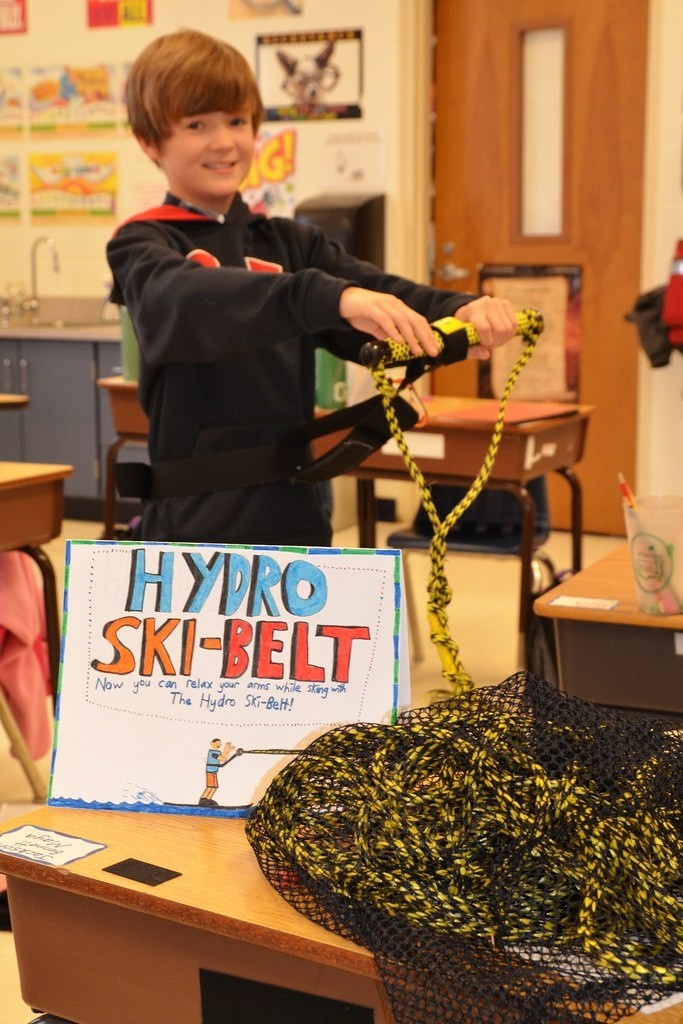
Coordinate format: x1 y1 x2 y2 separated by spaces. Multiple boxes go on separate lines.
13 236 61 324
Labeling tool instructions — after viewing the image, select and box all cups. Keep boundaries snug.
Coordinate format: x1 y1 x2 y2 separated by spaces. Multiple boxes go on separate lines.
620 494 683 616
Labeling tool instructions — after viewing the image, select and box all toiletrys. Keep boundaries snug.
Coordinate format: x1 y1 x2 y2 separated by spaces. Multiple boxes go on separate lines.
102 281 120 324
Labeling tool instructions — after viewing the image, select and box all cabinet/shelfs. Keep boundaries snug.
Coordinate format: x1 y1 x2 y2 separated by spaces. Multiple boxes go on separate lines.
0 337 98 499
95 340 150 502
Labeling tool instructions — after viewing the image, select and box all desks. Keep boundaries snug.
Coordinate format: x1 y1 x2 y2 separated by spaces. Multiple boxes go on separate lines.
533 541 683 731
96 376 597 674
0 804 683 1024
0 460 75 720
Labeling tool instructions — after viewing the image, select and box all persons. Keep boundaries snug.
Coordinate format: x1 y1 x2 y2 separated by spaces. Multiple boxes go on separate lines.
104 26 547 540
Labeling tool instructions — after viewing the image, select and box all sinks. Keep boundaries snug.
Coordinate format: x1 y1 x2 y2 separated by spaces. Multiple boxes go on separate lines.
8 320 120 328
